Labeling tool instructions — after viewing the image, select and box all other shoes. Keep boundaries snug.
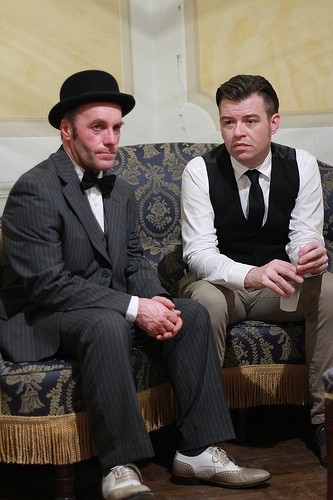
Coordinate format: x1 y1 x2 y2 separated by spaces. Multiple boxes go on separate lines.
316 424 328 468
103 463 154 500
172 446 272 489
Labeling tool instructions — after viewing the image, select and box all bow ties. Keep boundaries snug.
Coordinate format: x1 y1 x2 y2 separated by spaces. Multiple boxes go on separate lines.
81 170 116 197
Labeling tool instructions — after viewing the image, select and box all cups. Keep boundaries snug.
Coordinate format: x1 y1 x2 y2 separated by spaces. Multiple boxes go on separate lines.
280 276 303 311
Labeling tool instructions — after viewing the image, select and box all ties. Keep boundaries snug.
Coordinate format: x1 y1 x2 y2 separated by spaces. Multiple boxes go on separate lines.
245 169 265 228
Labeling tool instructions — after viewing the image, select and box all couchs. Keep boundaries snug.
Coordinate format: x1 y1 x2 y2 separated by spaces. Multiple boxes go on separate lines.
0 143 332 500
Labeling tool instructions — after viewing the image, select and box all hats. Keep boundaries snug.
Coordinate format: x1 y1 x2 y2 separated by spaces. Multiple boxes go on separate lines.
49 69 135 131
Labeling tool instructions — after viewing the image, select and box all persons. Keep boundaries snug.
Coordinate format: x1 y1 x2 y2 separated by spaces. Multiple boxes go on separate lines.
0 70 274 499
177 74 333 468
